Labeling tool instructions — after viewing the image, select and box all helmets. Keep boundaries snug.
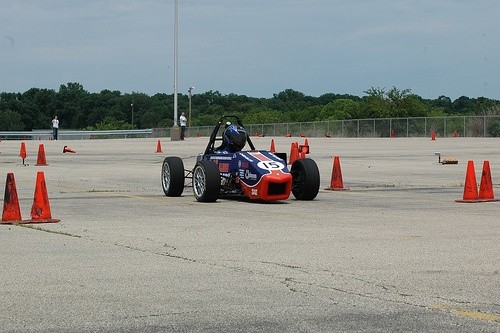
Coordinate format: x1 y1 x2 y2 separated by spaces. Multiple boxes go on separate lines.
222 124 247 151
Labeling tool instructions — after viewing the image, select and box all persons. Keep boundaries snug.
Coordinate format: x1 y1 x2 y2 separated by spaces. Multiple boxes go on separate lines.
180 111 187 140
212 125 247 153
51 115 59 140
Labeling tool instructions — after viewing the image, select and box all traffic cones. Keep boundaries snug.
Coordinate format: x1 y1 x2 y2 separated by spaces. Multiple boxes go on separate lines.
0 171 22 225
258 133 264 137
324 156 351 191
19 141 27 159
269 138 276 152
35 144 49 166
285 133 292 138
477 160 500 202
324 134 331 139
455 131 459 137
287 139 310 165
63 145 76 154
455 160 480 204
255 132 258 137
299 133 306 137
20 171 61 224
431 130 437 140
155 139 163 153
391 127 395 137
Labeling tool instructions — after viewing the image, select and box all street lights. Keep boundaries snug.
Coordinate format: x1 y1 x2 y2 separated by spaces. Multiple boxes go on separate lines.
188 86 194 128
